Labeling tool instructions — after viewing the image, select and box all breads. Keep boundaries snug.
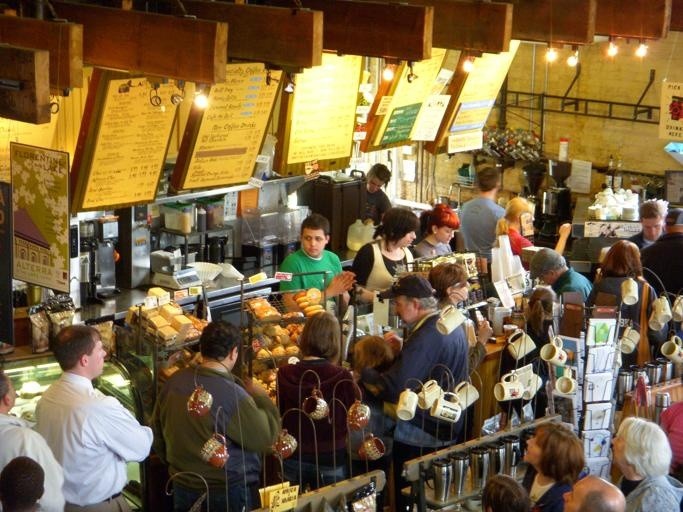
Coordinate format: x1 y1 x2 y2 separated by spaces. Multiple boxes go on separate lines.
124 288 209 352
244 289 326 407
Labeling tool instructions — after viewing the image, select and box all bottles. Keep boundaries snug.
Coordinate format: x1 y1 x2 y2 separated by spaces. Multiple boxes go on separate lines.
588 155 647 221
196 208 207 232
474 308 484 327
487 295 526 336
481 124 540 163
181 208 191 235
12 283 41 306
558 138 568 162
469 163 476 182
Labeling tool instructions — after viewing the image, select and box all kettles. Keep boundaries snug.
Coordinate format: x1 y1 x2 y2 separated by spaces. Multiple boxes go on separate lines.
205 236 228 263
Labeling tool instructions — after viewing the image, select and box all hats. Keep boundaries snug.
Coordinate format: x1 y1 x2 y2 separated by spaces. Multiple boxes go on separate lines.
380 274 435 299
529 248 557 279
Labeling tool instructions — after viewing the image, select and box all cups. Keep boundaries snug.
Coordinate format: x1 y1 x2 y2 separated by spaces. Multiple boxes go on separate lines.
302 390 328 419
271 428 297 460
396 329 579 503
357 434 384 461
436 304 465 335
489 236 523 281
187 387 213 418
200 433 229 469
346 400 370 432
618 278 682 422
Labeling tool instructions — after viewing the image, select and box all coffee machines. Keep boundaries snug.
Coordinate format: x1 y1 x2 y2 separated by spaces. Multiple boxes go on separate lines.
519 160 573 249
79 219 118 304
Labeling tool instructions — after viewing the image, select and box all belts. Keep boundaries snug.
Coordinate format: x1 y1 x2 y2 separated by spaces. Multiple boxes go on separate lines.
103 491 121 501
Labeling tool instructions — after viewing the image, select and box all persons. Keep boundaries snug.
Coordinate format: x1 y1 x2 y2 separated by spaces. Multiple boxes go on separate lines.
275 164 683 511
149 319 281 512
34 325 153 512
0 455 44 511
0 367 64 511
